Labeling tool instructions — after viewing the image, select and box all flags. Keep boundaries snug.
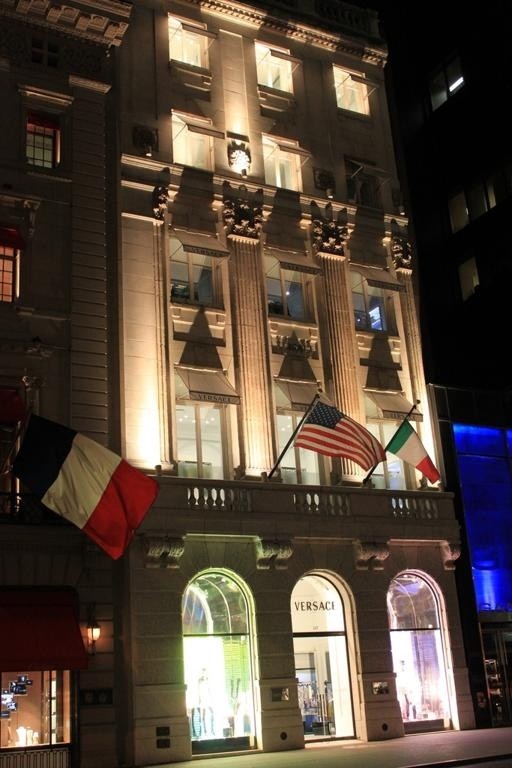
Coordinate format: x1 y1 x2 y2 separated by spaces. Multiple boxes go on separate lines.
291 400 388 473
10 410 159 561
387 417 443 484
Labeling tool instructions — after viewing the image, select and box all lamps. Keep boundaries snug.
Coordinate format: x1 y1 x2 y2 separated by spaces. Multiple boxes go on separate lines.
86 600 100 656
